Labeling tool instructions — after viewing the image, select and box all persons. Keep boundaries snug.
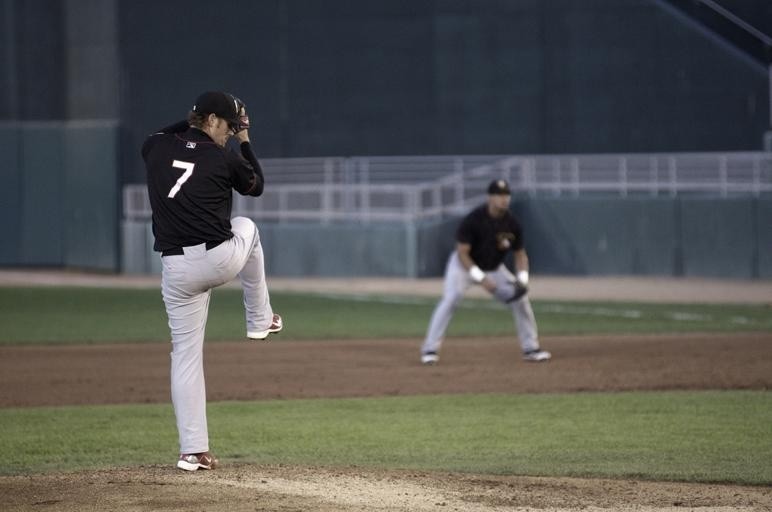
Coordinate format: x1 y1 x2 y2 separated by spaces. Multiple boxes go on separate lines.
420 178 551 365
142 91 282 471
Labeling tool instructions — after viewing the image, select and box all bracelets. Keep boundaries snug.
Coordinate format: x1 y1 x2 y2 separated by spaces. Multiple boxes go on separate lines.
518 270 529 283
469 264 485 283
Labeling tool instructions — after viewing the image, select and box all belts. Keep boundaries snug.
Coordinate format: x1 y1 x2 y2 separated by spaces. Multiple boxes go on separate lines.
161 238 224 259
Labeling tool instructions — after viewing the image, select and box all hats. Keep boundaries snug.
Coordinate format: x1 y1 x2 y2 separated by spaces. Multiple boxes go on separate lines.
191 91 240 129
485 178 515 195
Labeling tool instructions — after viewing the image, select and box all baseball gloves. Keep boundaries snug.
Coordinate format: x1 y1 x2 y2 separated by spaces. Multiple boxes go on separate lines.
507 281 525 302
225 92 249 132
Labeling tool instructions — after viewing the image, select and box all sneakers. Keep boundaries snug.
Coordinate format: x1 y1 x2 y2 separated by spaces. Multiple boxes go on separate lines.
176 450 220 473
419 352 440 366
247 313 284 341
523 350 553 361
